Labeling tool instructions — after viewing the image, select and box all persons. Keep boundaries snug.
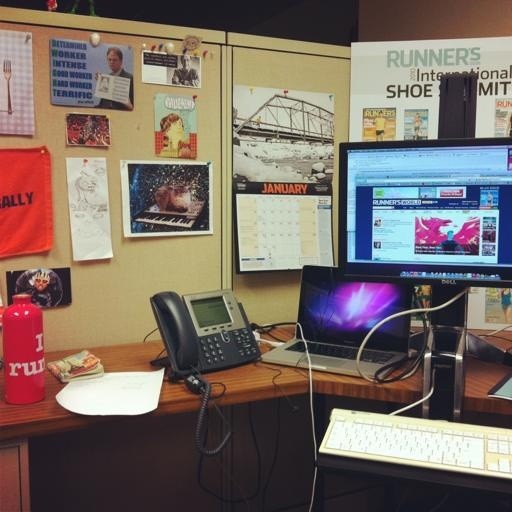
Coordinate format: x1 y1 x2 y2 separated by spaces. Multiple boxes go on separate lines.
92 46 134 111
413 111 424 140
373 110 388 141
170 54 201 89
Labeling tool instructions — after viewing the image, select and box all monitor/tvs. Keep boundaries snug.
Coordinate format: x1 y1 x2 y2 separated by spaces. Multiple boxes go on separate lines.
338 137 511 288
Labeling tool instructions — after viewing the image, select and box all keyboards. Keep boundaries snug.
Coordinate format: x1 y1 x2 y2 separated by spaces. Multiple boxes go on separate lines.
318 406 512 481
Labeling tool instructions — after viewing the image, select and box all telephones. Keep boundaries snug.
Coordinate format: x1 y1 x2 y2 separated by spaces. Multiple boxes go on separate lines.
150 289 262 376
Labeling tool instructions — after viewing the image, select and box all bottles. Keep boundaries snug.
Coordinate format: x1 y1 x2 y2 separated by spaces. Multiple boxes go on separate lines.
2 292 46 405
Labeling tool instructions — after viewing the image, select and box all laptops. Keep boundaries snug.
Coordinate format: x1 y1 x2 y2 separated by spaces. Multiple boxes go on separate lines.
260 264 411 380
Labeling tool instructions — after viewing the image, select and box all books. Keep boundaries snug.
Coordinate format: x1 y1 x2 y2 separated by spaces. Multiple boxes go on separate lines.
46 348 104 385
486 368 512 402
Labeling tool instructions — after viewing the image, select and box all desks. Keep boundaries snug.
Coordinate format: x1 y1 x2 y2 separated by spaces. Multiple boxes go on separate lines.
2 329 511 511
